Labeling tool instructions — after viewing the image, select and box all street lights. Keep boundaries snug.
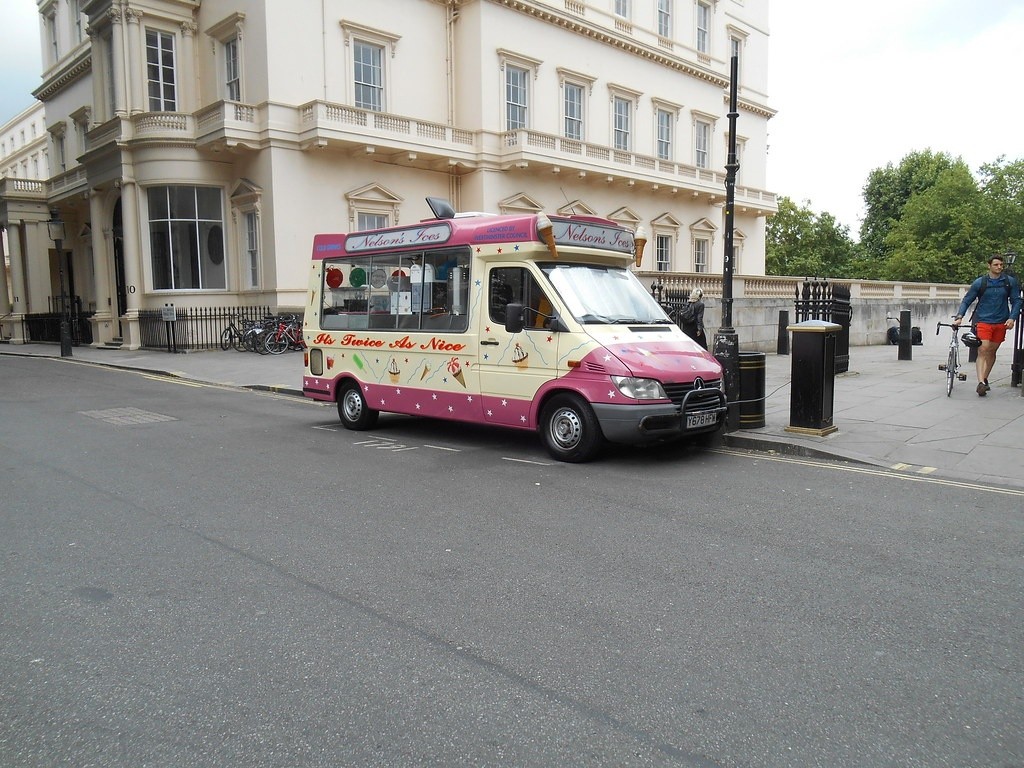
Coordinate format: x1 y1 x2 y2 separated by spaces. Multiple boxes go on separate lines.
1003 248 1018 276
46 205 73 356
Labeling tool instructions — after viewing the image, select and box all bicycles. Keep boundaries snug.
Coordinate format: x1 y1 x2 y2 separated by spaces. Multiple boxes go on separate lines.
936 316 982 397
220 310 307 355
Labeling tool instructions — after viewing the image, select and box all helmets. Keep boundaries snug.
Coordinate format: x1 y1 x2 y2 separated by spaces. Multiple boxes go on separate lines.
961 332 982 348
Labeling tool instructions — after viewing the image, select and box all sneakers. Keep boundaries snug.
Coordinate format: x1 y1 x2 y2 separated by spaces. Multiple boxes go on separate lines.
976 382 987 396
984 378 990 391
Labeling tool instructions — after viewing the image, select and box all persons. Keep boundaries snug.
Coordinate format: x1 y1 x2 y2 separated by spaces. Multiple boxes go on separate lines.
952 255 1020 396
684 289 707 349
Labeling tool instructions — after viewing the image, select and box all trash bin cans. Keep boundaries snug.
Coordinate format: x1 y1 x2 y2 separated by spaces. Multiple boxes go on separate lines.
738 351 766 429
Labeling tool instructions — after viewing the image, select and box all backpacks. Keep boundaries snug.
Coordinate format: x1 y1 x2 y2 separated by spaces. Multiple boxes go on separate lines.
680 300 700 324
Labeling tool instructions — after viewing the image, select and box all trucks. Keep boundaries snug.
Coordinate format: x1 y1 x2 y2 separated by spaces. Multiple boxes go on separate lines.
300 198 730 464
